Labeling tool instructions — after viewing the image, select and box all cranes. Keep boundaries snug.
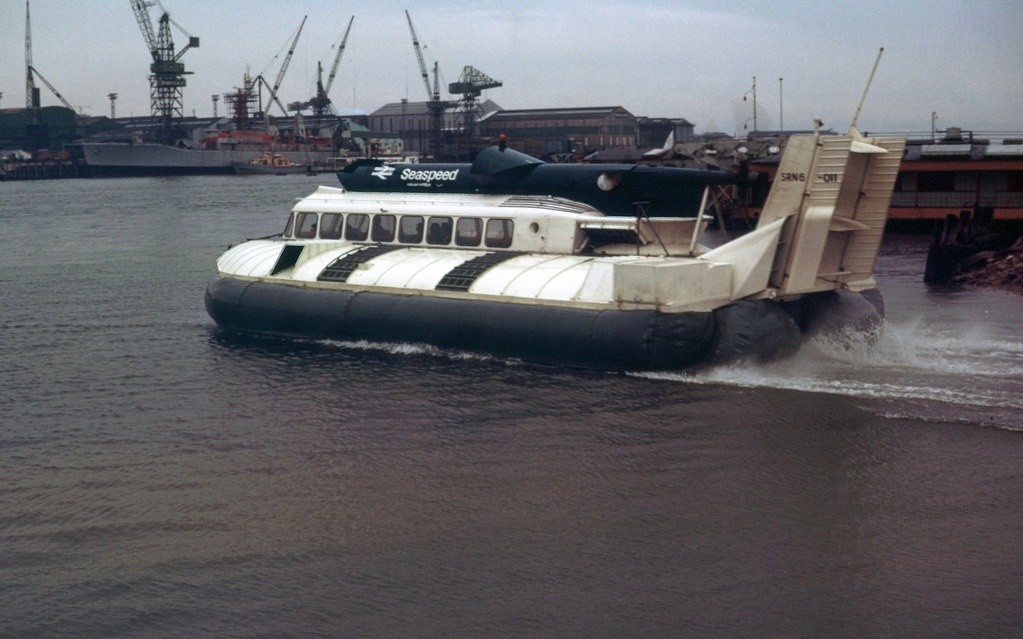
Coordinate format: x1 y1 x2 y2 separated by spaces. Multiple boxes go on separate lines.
450 64 504 164
132 0 199 144
23 0 38 109
286 14 356 144
403 9 461 162
222 14 309 134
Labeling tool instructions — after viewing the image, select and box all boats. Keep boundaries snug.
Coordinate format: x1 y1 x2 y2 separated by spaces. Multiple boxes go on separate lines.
231 150 308 176
81 140 435 179
203 39 909 381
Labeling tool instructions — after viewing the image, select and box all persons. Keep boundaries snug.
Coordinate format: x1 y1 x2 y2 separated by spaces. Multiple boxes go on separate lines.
338 220 358 240
415 222 452 244
177 140 186 149
364 215 384 240
309 223 323 238
